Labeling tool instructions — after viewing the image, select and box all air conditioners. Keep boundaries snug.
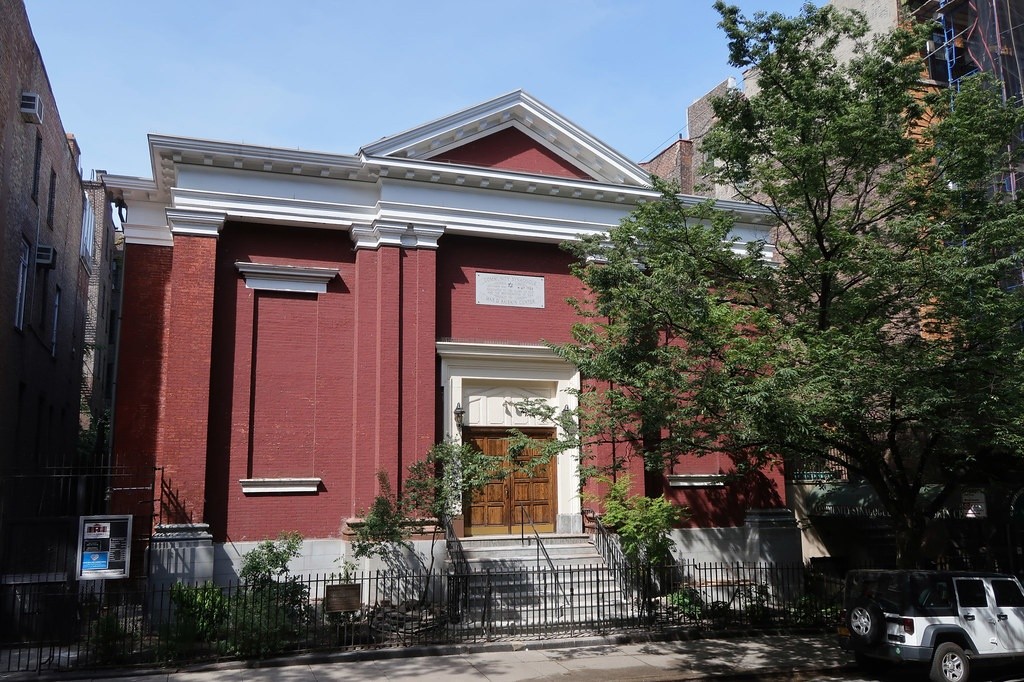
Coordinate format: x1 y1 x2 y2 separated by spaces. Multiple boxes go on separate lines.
36 245 57 270
20 92 44 124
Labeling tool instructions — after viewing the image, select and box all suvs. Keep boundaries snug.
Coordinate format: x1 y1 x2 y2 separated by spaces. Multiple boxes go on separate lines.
836 569 1024 682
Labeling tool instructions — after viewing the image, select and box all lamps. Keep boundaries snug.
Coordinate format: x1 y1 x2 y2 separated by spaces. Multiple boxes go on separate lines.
454 402 465 433
562 404 571 431
